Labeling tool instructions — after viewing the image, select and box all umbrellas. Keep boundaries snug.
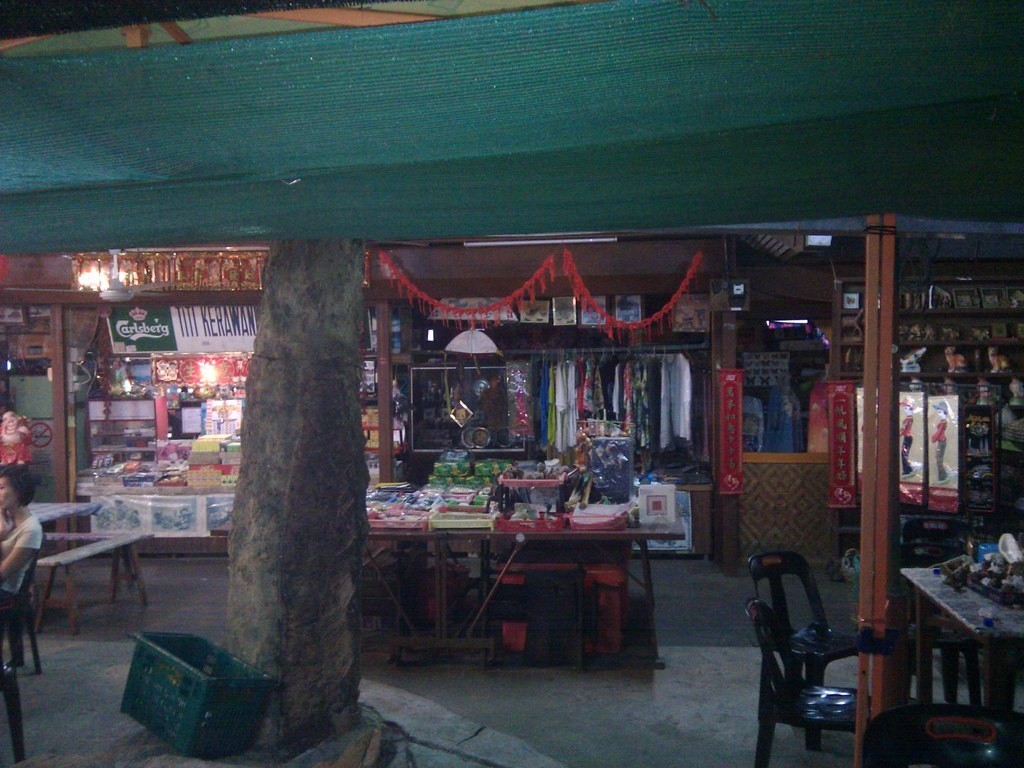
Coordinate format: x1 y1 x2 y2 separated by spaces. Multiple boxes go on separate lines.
445 329 499 354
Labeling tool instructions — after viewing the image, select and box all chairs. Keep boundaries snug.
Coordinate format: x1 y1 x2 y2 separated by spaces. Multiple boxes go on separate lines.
896 540 983 706
0 591 32 763
745 595 873 768
748 548 861 750
863 699 1023 768
901 516 976 547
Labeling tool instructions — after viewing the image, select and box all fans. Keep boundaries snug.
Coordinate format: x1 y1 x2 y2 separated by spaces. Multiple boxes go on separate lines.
3 247 177 303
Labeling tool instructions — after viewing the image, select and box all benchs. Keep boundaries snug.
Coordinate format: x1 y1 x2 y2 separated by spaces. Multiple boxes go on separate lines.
33 531 146 636
37 532 156 595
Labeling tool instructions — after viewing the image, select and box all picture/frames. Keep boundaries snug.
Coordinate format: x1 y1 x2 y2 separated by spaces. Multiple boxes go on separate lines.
980 287 1008 308
1005 286 1024 308
671 294 711 332
551 296 577 326
611 293 647 328
841 291 861 310
517 297 552 327
577 295 610 330
952 287 981 309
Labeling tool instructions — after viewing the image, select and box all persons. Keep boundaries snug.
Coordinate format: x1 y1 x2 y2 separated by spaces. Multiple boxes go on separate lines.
0 464 43 608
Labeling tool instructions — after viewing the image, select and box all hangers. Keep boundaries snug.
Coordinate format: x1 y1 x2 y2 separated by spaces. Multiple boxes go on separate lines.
539 344 697 369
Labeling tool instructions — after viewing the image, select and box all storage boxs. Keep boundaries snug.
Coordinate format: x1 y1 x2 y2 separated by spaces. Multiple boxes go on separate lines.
365 463 634 656
94 433 242 490
119 630 277 759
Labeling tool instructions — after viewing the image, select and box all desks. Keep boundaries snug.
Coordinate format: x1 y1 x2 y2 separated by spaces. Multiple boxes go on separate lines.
209 507 687 672
27 502 103 523
898 567 1024 712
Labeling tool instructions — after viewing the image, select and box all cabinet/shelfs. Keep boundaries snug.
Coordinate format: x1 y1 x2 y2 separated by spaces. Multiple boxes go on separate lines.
86 395 169 466
832 274 1024 568
